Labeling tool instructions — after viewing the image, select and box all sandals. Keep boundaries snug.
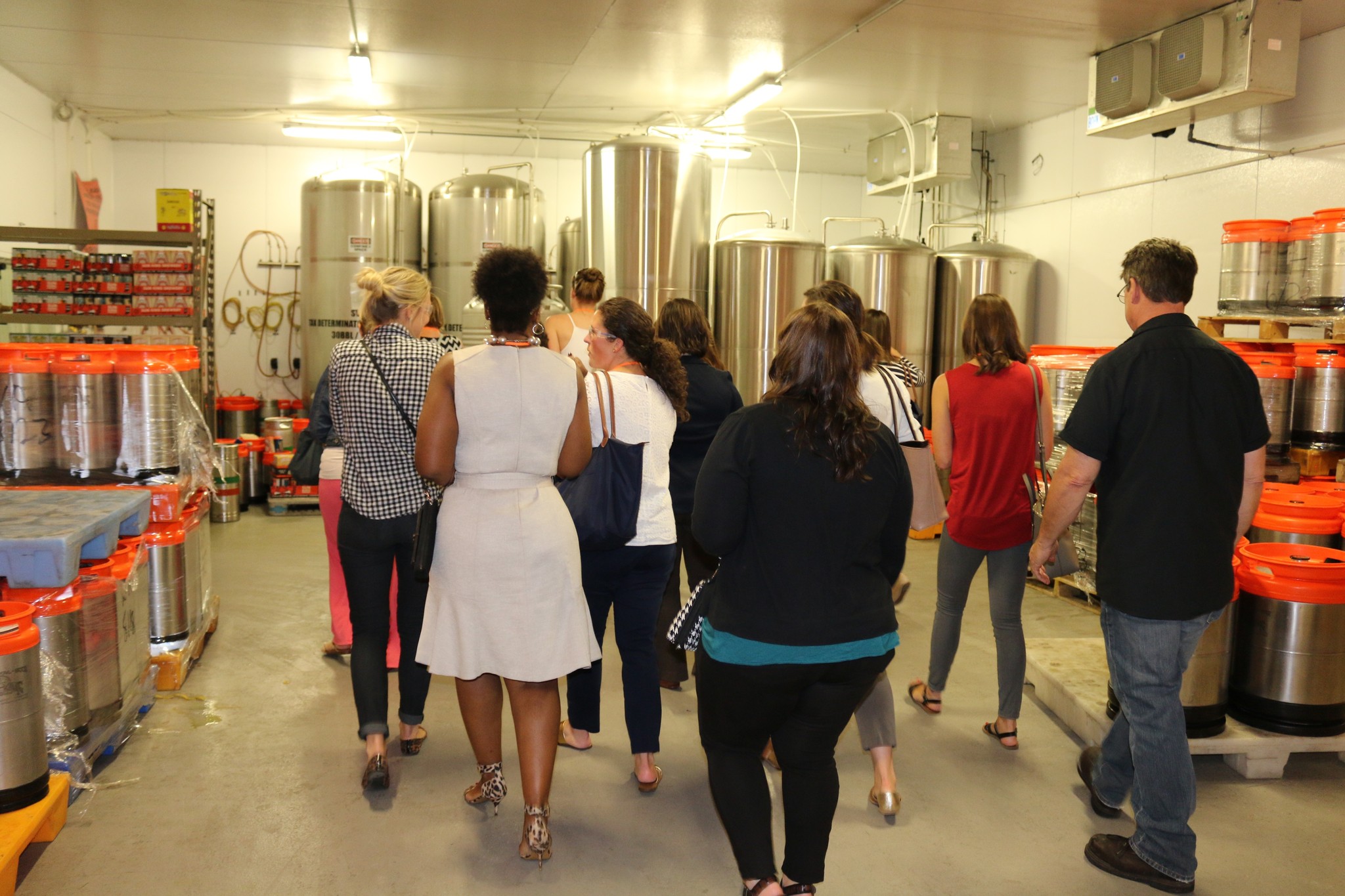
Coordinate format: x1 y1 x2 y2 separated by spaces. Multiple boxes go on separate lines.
907 682 941 714
743 875 782 896
983 722 1019 749
778 875 816 896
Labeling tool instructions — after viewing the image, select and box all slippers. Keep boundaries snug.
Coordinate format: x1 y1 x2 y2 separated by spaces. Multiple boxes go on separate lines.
558 721 592 751
634 764 662 791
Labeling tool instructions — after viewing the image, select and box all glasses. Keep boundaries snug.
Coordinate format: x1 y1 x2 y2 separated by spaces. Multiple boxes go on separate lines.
1118 277 1136 303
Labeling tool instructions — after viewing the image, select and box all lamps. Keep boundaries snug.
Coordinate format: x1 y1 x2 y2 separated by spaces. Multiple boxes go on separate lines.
347 51 373 94
281 124 401 143
701 78 783 128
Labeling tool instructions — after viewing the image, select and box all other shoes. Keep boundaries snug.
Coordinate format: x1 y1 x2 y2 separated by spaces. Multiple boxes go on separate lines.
762 745 783 771
399 726 427 753
869 786 900 816
324 640 352 656
361 754 389 789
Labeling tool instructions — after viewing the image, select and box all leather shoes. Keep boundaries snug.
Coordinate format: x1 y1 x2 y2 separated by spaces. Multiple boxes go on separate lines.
1084 834 1195 893
1077 745 1122 818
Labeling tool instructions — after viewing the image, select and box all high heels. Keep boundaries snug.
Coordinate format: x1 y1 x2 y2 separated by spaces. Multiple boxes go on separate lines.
465 761 506 817
519 804 553 869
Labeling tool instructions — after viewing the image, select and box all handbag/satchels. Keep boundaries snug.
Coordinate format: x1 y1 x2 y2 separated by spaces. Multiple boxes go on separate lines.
411 469 451 572
555 368 648 547
290 428 322 482
875 365 949 532
1022 470 1079 579
667 559 723 650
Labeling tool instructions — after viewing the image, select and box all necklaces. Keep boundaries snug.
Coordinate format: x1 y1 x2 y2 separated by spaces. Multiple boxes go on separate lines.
483 335 541 346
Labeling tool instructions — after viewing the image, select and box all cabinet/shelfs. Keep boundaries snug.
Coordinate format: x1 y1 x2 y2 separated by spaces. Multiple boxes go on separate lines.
0 187 216 446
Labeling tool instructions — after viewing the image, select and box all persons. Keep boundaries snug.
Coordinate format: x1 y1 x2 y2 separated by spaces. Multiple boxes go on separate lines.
1029 238 1271 894
654 298 743 686
862 308 926 388
310 309 400 667
761 281 924 825
328 265 448 791
557 297 690 790
908 293 1054 750
420 292 462 352
544 267 606 373
414 246 603 871
691 302 913 896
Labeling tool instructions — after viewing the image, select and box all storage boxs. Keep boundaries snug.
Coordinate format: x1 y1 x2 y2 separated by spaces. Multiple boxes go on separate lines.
8 246 208 394
234 433 317 497
155 188 194 232
